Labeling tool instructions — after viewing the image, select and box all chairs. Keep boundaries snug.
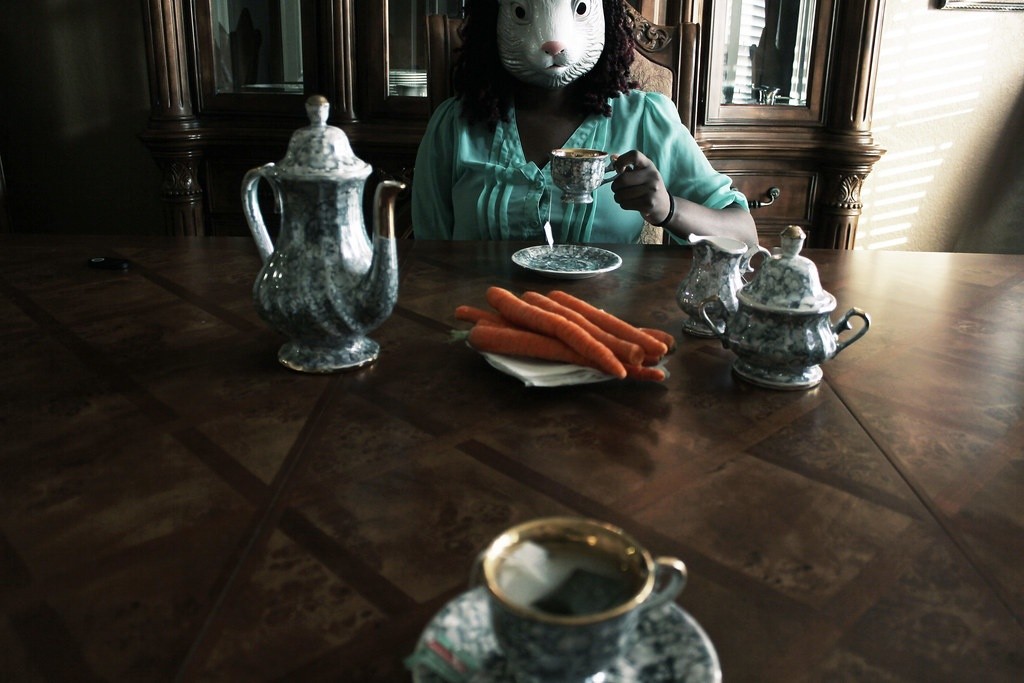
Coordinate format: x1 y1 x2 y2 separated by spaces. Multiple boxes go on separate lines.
426 0 700 245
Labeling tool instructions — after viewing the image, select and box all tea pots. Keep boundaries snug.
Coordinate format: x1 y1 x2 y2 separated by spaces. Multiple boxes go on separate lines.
240 96 406 373
698 225 872 391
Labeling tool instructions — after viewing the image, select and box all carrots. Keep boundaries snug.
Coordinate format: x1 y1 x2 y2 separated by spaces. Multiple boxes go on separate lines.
455 286 675 381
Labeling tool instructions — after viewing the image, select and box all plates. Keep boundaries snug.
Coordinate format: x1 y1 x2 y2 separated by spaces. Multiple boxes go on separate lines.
510 245 623 279
410 586 722 683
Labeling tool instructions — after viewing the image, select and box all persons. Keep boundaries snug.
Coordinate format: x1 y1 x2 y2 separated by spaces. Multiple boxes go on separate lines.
411 0 758 250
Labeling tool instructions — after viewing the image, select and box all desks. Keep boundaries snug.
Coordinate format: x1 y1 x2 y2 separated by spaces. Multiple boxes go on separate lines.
0 231 1024 683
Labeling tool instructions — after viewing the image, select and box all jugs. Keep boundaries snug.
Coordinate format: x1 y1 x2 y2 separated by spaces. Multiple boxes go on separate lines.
675 233 771 338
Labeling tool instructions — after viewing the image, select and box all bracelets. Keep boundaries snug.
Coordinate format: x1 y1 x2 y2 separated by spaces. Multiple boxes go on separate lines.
654 191 675 226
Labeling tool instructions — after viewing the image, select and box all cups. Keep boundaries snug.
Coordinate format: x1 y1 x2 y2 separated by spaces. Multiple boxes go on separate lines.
551 148 625 204
480 516 687 683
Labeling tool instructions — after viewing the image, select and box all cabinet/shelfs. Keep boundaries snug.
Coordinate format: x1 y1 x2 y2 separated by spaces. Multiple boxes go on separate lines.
145 0 881 247
